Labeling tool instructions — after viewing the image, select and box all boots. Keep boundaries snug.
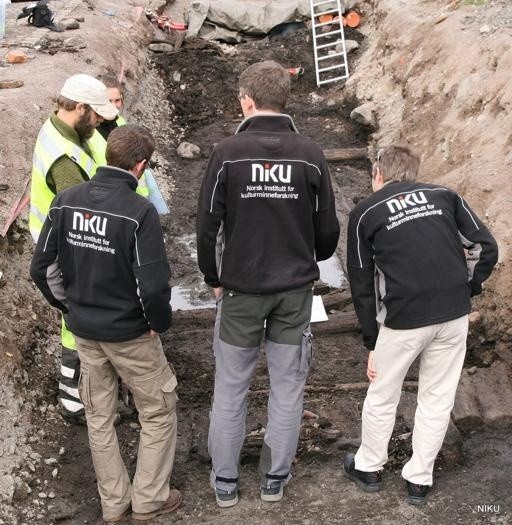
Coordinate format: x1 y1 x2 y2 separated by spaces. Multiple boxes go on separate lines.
101 489 182 522
407 482 428 505
210 468 239 508
58 346 132 425
260 471 292 501
343 453 383 492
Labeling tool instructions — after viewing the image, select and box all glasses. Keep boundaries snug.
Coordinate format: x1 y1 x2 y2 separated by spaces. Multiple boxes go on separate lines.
236 93 247 101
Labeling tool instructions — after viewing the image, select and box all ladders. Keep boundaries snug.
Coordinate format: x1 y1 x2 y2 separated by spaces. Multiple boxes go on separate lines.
310 0 349 88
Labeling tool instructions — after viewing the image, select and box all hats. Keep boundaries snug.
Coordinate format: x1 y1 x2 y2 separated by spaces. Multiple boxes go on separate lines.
61 74 119 117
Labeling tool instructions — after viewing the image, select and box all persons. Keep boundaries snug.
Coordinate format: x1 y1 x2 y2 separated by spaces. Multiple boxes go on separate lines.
28 75 134 428
29 123 184 525
346 143 500 508
194 60 342 511
96 72 150 199
